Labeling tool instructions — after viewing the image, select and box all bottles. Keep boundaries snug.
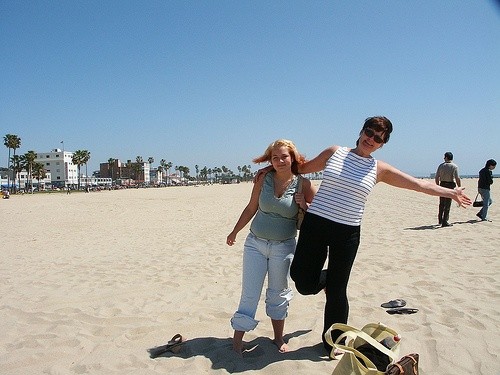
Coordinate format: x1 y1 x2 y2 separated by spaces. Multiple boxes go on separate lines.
379 333 403 349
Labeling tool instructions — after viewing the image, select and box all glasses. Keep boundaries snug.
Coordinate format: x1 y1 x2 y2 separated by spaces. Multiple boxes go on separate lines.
364 128 385 144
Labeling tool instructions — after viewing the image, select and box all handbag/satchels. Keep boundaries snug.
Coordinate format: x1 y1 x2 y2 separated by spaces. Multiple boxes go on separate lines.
325 323 402 375
473 193 484 207
385 354 419 375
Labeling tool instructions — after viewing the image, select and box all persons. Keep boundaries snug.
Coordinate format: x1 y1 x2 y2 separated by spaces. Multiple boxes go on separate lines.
478 159 498 222
2 181 192 199
193 174 254 186
435 151 462 228
224 138 317 355
253 116 472 356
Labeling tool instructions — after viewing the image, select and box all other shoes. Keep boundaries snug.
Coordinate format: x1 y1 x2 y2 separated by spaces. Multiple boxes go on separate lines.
439 220 442 224
442 223 453 227
477 213 482 219
482 219 492 222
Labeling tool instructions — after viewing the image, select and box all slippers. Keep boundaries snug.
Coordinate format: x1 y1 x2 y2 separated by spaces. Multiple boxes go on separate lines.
150 334 187 358
386 308 419 314
381 299 406 307
